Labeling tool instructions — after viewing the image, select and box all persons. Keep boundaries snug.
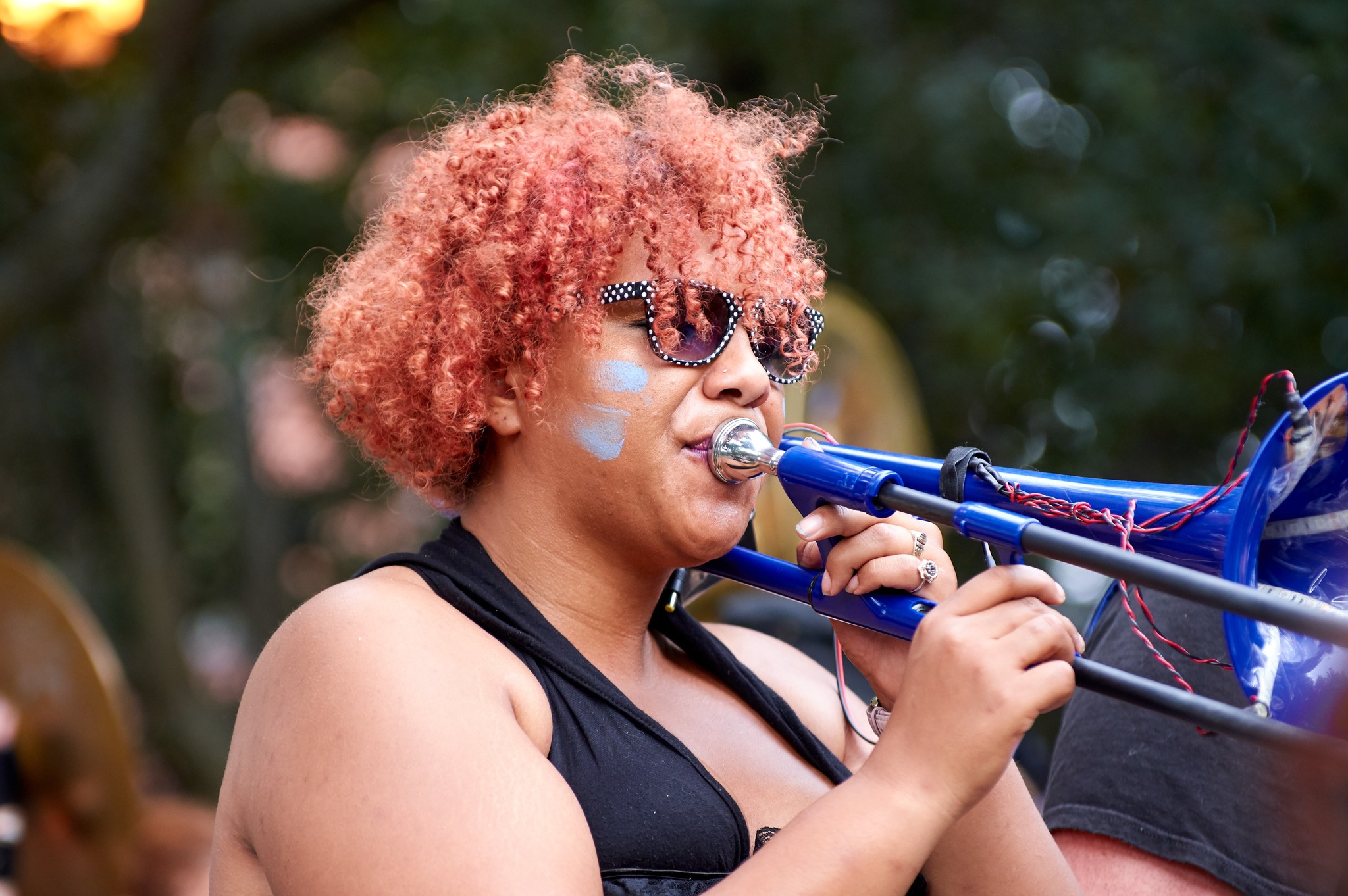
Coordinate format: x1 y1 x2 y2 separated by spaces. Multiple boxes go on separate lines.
1 55 1347 896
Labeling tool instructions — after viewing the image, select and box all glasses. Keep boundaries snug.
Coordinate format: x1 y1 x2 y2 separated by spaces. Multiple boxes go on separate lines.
589 275 824 384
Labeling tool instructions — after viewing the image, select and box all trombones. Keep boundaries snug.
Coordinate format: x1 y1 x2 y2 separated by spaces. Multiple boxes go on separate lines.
707 369 1347 761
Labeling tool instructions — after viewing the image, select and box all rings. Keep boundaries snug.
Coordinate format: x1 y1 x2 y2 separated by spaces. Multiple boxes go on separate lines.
909 561 937 593
907 530 928 556
